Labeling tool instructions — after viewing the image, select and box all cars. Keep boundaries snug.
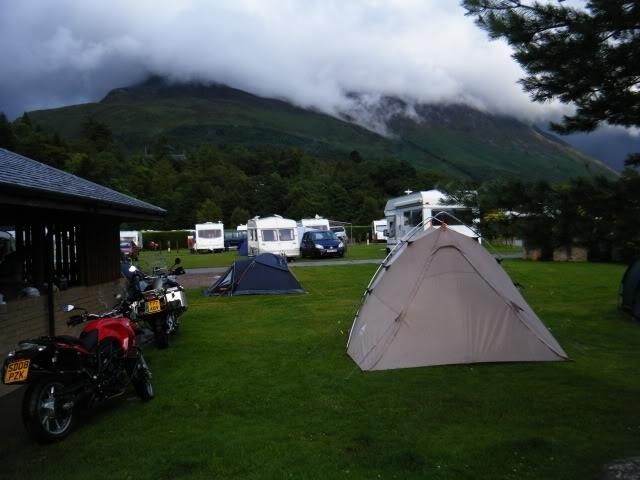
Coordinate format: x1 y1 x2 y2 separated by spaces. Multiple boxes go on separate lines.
300 230 346 258
120 240 142 262
144 240 164 251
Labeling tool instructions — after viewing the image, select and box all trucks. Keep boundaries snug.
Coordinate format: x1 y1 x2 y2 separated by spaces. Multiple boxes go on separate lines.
382 186 484 259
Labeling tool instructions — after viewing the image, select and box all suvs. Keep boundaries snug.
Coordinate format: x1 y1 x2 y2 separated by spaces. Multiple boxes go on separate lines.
330 226 350 243
224 230 250 250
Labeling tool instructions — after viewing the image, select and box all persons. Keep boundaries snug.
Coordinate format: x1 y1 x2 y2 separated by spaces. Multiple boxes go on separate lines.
21 280 40 297
365 233 370 246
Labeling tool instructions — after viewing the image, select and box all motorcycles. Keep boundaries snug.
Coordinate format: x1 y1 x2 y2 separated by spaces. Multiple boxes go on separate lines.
2 288 155 446
118 255 193 348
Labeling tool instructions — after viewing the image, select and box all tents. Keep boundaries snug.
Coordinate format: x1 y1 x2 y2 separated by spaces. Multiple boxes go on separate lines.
203 253 307 298
347 211 573 375
617 259 640 322
